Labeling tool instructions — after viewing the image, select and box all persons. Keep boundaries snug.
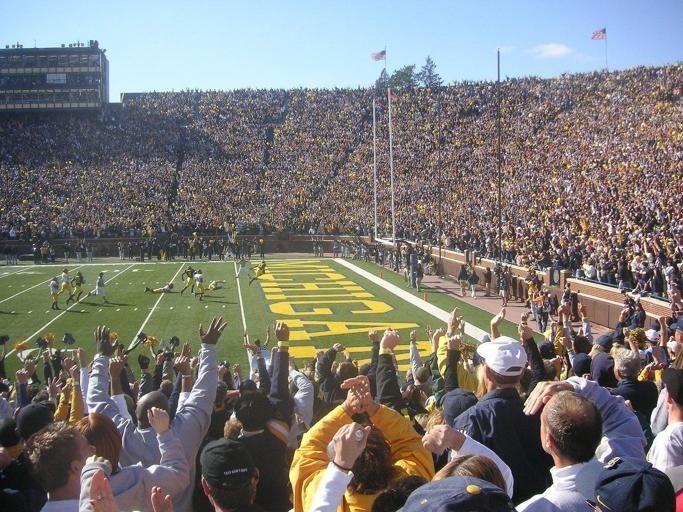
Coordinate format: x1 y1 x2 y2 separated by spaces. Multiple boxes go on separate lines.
144 265 227 301
234 256 267 286
0 85 401 276
49 268 109 308
0 307 681 511
402 61 683 308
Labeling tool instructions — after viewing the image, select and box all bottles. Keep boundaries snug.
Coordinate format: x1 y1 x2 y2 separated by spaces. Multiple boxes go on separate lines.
325 428 363 462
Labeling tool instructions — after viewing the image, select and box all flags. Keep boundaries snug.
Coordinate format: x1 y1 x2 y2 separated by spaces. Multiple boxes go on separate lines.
371 49 385 61
591 28 605 41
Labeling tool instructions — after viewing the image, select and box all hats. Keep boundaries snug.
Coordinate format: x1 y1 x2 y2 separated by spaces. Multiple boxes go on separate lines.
476 335 528 378
199 437 257 489
538 341 555 355
440 388 479 414
670 316 683 332
661 366 683 402
573 353 592 374
666 341 682 352
394 475 511 512
644 329 660 342
593 457 677 512
595 335 613 346
16 402 55 437
